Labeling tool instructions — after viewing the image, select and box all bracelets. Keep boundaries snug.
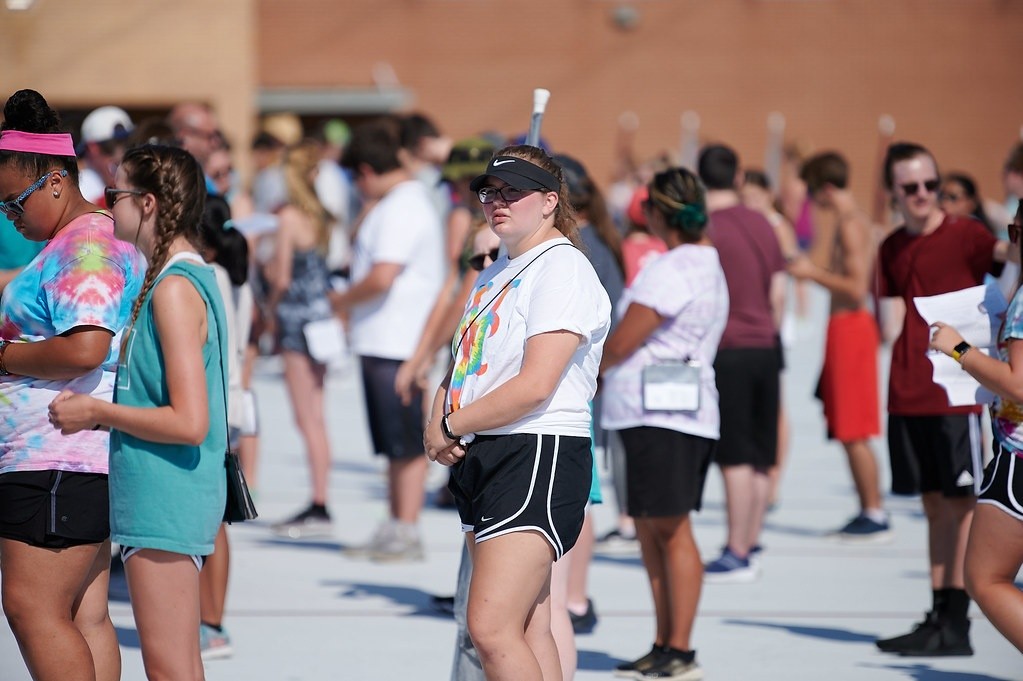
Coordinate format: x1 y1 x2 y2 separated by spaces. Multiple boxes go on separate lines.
91 424 103 432
0 339 14 375
960 346 980 370
950 340 970 363
442 413 459 440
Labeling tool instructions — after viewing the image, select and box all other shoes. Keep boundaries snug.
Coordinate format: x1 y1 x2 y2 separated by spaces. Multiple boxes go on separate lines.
567 599 597 636
591 530 641 554
286 504 424 564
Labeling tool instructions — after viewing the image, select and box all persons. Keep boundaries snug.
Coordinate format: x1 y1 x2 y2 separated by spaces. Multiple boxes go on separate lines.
1 87 1022 681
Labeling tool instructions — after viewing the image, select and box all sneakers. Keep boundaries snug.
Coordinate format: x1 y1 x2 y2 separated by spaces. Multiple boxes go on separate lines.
614 643 699 681
877 611 973 656
200 624 232 661
840 513 889 535
702 545 764 584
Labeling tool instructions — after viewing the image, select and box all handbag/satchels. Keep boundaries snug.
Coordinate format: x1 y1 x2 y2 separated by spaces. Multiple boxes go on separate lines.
774 331 786 369
304 314 347 365
642 356 700 412
223 452 258 525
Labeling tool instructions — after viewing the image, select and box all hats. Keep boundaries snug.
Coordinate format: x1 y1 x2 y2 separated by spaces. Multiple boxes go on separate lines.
469 155 562 198
263 113 349 146
629 194 650 225
75 105 135 155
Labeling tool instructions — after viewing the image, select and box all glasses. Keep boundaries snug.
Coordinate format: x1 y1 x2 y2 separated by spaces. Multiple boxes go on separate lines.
477 185 547 204
894 179 940 195
939 191 958 201
467 248 499 271
104 185 147 209
0 170 67 216
1007 224 1022 243
